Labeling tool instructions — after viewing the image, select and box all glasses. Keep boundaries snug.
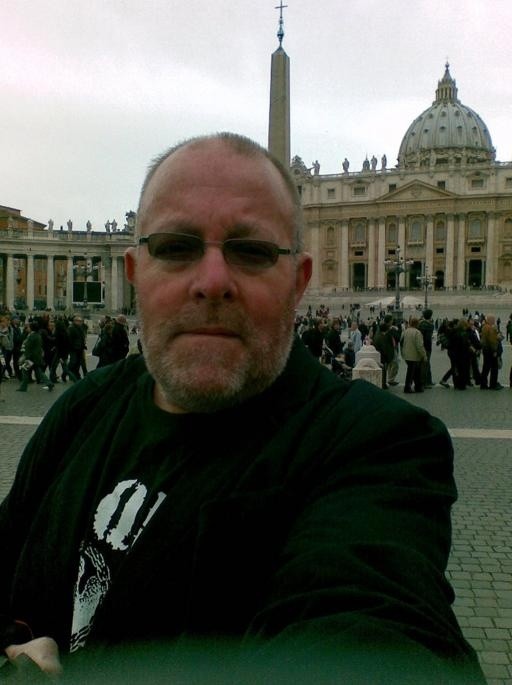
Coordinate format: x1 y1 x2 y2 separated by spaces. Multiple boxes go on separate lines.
139 232 298 269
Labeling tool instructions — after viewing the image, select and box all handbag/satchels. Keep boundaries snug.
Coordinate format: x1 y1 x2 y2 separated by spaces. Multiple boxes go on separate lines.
92 336 99 357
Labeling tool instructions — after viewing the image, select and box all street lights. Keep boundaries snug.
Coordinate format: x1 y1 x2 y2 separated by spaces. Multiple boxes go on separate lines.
74 259 99 308
383 247 413 309
415 263 437 309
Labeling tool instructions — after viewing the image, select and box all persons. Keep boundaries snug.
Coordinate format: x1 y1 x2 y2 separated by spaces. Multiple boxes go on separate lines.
436 308 510 390
399 318 428 392
310 158 321 175
417 310 434 386
44 216 118 234
96 314 129 370
0 308 87 393
295 304 409 389
0 133 487 684
338 147 470 172
